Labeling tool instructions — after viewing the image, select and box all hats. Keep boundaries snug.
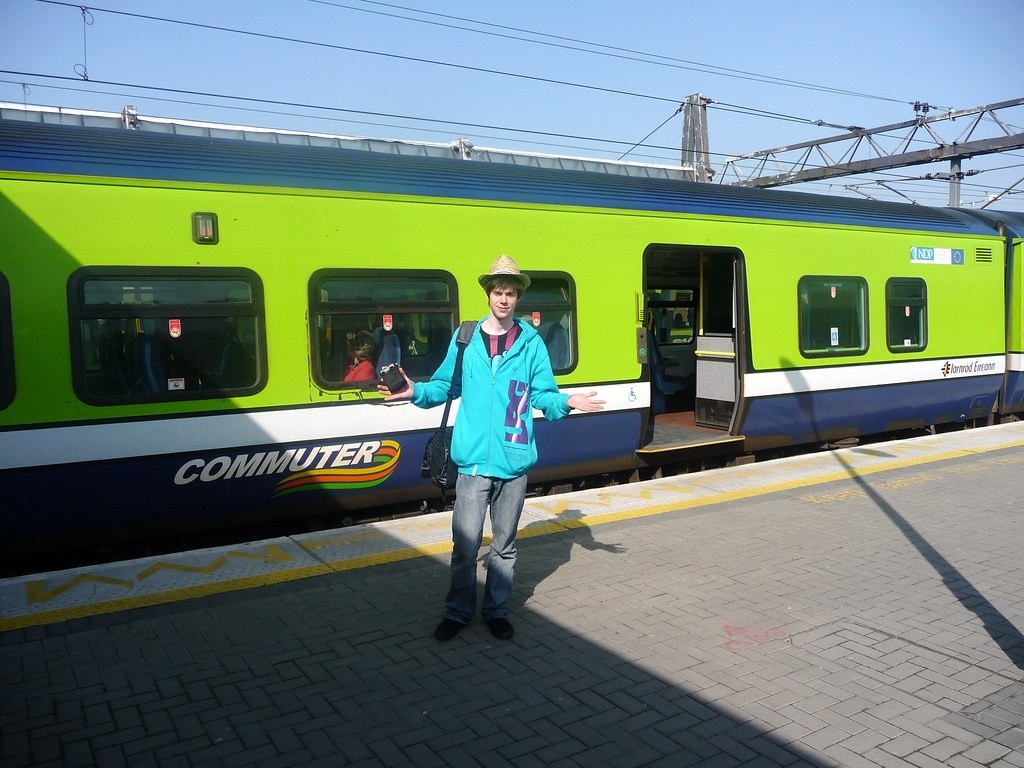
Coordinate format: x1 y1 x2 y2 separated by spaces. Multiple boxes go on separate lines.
349 329 375 359
478 255 531 293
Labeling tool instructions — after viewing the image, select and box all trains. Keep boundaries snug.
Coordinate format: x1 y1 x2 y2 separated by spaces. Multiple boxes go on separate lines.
0 95 1024 561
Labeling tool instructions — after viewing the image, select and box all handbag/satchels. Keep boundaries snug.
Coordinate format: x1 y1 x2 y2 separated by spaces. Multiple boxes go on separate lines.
420 426 458 490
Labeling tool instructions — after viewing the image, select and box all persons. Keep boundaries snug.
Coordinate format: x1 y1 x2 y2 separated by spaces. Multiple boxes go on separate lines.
338 328 376 382
98 317 131 392
377 256 607 642
672 312 687 329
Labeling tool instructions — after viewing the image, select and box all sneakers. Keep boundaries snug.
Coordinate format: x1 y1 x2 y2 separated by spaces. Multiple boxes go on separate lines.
435 616 465 641
485 618 513 640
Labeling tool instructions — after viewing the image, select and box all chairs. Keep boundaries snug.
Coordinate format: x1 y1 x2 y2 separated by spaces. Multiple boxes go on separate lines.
335 326 405 383
97 330 170 395
543 322 571 371
